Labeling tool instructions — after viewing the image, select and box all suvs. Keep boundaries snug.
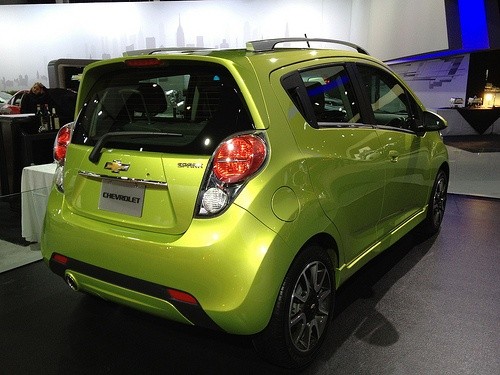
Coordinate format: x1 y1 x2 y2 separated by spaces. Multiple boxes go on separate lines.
39 38 452 366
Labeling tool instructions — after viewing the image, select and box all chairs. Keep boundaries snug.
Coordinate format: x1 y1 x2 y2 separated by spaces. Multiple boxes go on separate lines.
135 83 167 116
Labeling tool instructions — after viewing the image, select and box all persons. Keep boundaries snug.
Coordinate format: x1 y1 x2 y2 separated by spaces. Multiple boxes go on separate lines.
20 81 48 115
